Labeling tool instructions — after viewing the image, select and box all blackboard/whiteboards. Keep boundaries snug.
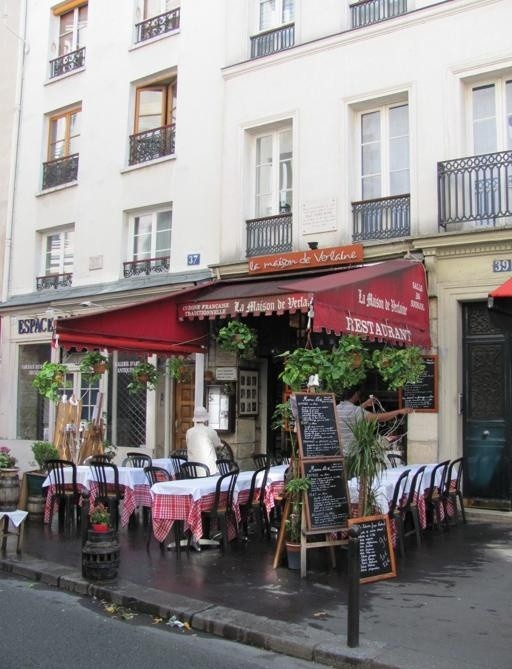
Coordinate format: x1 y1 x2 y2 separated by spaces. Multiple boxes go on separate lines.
292 391 343 460
348 514 397 584
300 457 353 530
398 355 439 412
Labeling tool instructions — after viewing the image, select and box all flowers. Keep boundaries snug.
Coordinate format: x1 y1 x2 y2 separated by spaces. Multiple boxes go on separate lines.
87 503 112 520
0 447 17 468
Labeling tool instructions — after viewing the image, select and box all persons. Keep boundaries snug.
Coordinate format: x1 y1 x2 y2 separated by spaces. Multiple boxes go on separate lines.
184 406 223 477
331 383 414 480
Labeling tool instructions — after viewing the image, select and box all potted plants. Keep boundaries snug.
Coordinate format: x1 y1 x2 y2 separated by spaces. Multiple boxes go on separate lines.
25 440 60 500
272 402 313 570
32 350 190 402
215 321 427 391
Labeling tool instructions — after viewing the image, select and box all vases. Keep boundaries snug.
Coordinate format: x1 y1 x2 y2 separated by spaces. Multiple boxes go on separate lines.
0 468 21 478
92 523 111 532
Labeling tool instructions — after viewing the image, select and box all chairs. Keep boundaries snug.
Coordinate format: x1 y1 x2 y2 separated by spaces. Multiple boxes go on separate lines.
143 459 241 558
251 453 292 473
195 466 292 559
389 457 467 560
352 453 409 474
42 442 235 539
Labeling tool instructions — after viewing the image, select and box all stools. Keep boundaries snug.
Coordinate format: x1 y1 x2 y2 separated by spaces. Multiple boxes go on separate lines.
0 512 25 560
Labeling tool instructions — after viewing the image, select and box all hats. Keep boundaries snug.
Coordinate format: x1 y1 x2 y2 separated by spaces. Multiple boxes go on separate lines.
192 407 210 422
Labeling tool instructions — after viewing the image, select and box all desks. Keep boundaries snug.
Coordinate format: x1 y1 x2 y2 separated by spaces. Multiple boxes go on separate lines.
329 462 463 551
